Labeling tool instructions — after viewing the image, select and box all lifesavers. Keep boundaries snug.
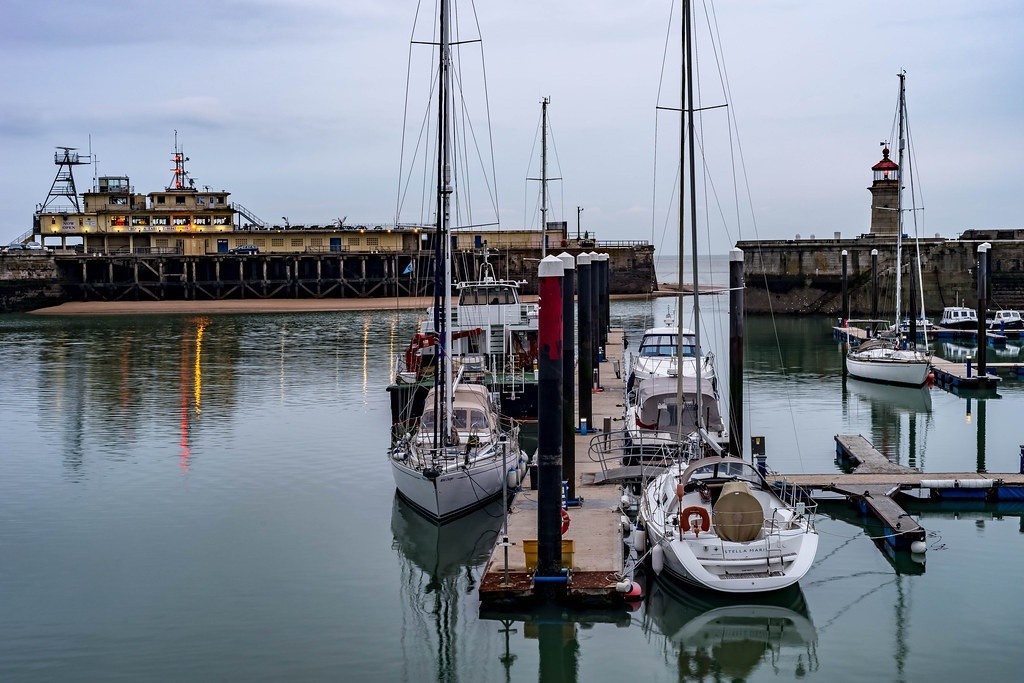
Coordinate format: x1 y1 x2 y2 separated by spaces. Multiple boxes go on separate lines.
682 506 710 531
561 509 570 534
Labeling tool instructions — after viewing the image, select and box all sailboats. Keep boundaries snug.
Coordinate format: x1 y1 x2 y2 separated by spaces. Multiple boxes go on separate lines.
639 581 824 683
386 1 838 598
941 341 980 365
389 487 515 683
845 373 933 468
844 67 941 390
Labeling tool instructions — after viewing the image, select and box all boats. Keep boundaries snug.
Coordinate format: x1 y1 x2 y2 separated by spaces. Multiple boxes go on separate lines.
986 308 1024 330
938 289 980 329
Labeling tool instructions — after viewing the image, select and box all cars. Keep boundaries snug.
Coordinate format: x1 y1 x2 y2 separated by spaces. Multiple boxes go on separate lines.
0 241 56 254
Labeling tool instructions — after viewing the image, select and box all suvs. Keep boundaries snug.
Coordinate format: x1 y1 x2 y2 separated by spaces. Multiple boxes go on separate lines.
229 244 259 256
